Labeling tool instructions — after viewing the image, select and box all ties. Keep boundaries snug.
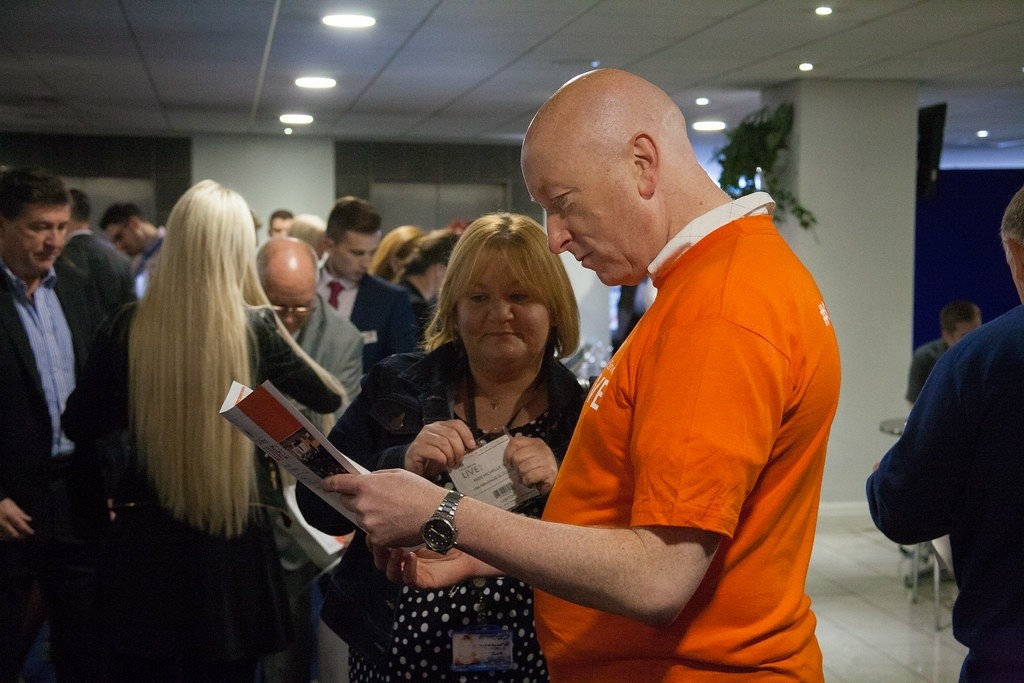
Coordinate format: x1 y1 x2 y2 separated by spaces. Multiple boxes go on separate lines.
327 281 346 308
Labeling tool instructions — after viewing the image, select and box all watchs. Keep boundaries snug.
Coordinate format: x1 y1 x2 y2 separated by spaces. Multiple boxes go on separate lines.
423 490 463 556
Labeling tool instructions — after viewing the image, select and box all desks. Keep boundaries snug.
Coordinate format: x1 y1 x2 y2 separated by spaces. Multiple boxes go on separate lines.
878 417 941 631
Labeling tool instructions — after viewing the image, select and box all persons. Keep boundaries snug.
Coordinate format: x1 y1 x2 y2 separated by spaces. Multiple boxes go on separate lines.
296 211 590 683
454 635 481 664
866 185 1023 683
0 165 467 683
907 300 981 413
320 70 840 683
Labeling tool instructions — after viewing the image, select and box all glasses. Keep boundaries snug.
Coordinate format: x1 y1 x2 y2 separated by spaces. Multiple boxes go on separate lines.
272 303 317 318
109 225 126 244
